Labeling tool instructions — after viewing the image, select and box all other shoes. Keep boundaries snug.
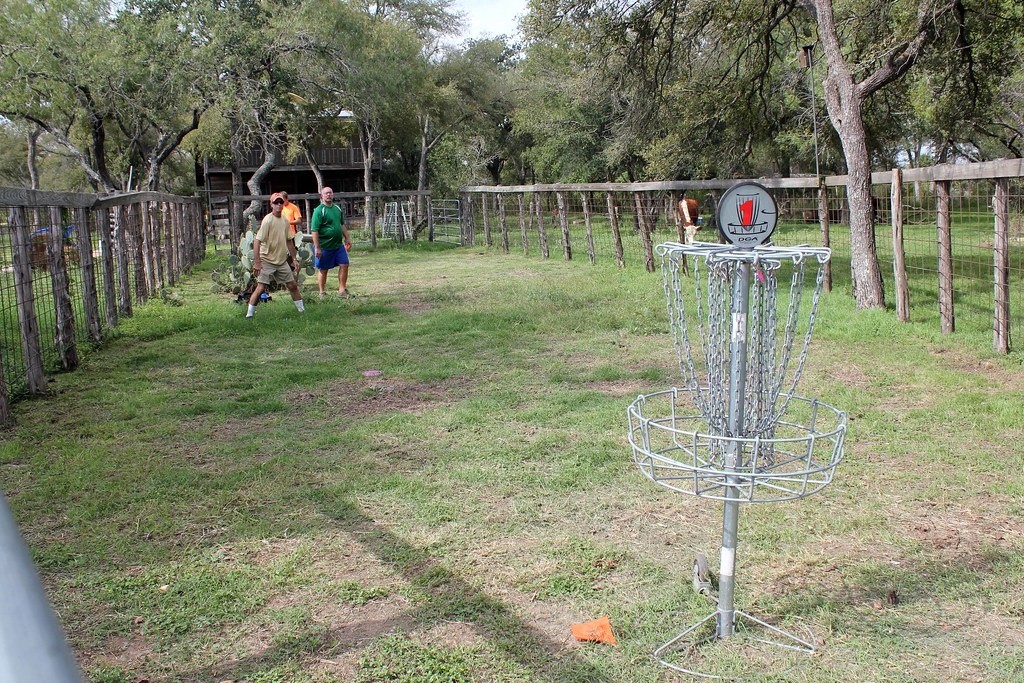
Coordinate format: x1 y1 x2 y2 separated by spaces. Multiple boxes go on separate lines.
336 288 355 301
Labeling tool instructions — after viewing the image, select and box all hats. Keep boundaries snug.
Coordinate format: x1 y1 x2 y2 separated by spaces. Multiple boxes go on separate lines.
270 193 284 204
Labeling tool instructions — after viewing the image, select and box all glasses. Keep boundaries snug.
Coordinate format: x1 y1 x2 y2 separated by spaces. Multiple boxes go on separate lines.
272 201 284 205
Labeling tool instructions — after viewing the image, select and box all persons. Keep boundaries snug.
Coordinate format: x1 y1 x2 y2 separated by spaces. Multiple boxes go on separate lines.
552 205 560 228
246 191 309 316
311 187 355 298
280 192 303 269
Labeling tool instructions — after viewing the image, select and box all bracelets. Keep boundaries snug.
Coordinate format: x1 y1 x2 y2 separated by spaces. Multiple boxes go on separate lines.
293 258 297 260
